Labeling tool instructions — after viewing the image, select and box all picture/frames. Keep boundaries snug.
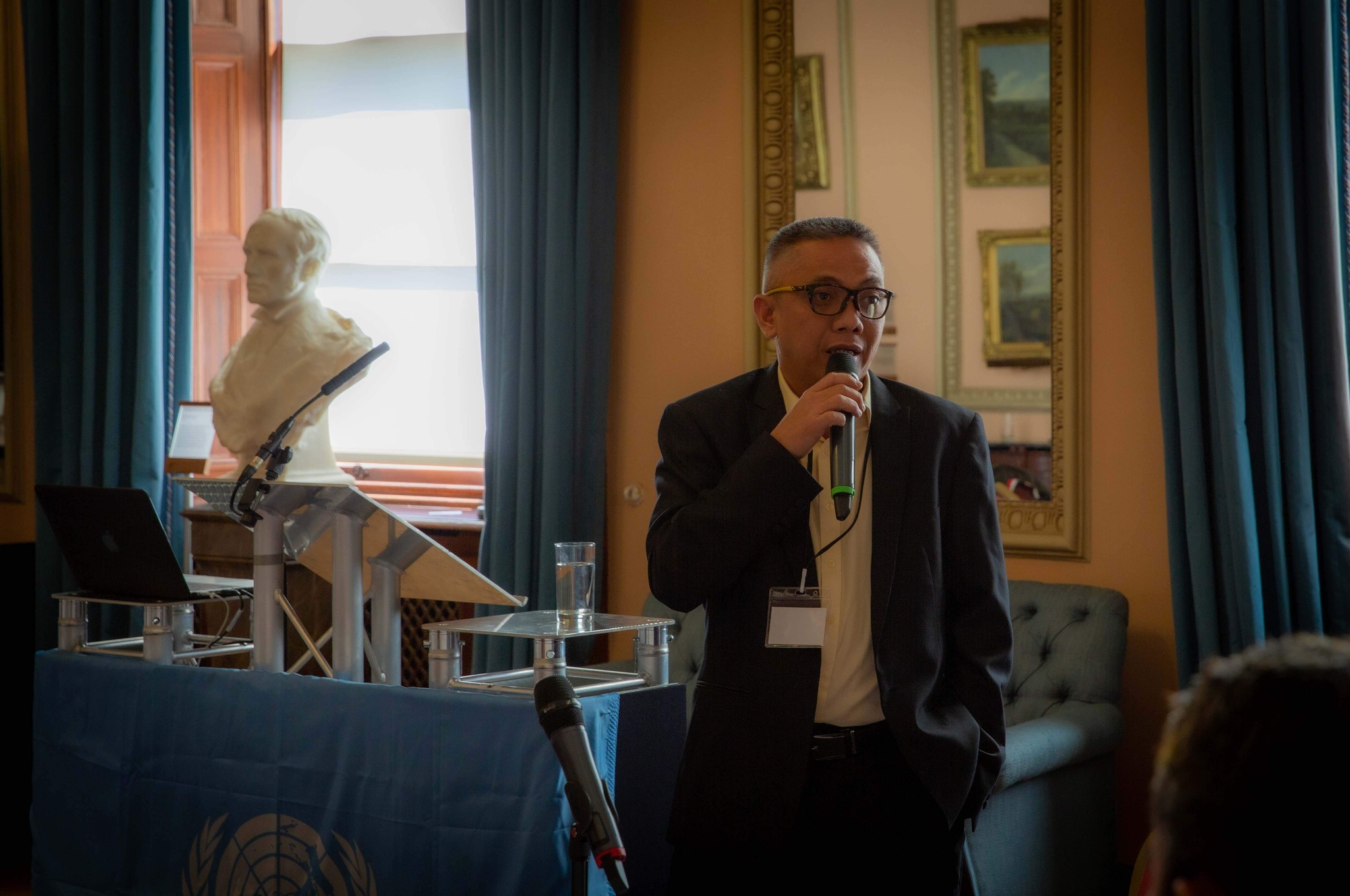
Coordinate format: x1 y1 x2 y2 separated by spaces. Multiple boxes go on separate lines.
962 17 1050 188
977 227 1053 369
790 54 829 190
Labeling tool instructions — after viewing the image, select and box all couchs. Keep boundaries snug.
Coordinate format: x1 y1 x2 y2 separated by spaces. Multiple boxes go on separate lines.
958 578 1128 896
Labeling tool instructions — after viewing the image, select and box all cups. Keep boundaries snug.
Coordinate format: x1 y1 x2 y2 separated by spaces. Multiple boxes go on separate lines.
554 542 596 624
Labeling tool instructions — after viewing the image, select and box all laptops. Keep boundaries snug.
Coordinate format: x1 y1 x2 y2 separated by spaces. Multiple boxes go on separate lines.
35 484 254 602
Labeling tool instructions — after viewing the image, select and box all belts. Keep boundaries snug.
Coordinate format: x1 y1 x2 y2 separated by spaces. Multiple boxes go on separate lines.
812 722 887 760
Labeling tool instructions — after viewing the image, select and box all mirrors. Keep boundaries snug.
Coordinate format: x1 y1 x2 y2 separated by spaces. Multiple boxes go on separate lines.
756 1 1091 559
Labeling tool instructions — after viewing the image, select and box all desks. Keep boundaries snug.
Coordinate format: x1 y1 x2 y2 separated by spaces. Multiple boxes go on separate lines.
50 574 254 671
419 610 675 702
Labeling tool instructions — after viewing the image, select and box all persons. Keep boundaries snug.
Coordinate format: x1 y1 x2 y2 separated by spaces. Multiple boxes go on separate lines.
211 207 369 462
644 218 1014 896
1128 635 1350 896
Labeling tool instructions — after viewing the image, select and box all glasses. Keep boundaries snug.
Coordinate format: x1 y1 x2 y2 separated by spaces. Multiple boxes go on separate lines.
763 283 895 320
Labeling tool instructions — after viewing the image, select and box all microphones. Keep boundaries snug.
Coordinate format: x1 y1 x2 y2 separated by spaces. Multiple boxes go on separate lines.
235 341 391 485
533 673 632 896
825 352 861 522
